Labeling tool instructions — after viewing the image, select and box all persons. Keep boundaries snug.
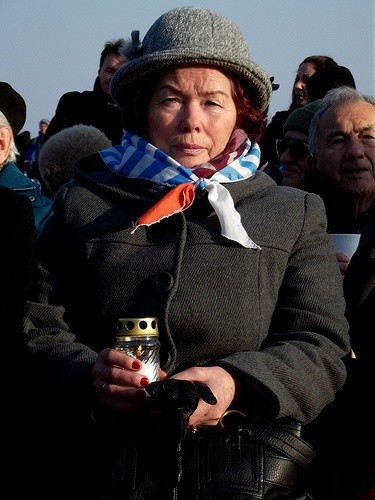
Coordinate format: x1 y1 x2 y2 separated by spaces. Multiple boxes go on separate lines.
0 5 375 500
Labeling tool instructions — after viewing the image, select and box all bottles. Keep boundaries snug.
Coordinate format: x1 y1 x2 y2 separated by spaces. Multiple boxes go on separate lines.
113 317 161 398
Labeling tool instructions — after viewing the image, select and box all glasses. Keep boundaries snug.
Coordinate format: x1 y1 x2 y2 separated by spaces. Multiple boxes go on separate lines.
275 139 310 161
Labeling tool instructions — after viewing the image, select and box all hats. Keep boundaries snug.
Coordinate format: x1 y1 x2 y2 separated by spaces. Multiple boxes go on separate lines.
283 99 322 136
109 5 271 119
0 82 26 138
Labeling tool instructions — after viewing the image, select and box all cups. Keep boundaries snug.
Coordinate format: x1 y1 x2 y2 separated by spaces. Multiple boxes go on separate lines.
328 234 361 279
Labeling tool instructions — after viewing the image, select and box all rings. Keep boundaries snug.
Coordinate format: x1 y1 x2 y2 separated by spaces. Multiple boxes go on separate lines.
100 383 106 391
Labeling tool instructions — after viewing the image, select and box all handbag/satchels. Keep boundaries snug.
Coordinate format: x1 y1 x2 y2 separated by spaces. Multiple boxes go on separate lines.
112 410 316 500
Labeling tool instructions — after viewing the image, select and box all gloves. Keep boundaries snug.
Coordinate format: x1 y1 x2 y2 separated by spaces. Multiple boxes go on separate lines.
145 378 218 414
